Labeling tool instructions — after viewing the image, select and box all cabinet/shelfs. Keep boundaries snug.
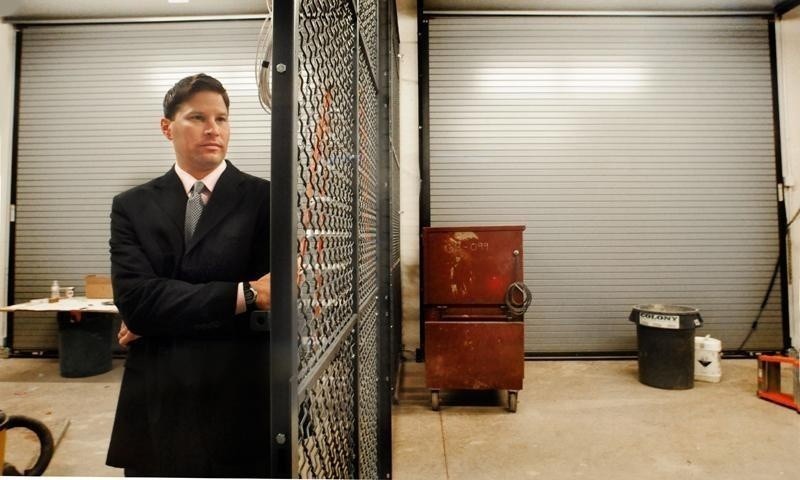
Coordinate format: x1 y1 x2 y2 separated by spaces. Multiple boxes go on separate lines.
423 224 526 414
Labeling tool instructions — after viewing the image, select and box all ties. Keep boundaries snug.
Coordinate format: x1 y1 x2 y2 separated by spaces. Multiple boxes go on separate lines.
184 182 205 249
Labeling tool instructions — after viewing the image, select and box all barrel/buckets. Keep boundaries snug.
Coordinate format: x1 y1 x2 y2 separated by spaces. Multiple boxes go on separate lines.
694 335 722 383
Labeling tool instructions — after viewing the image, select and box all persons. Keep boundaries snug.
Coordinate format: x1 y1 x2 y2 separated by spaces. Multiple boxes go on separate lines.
105 73 271 477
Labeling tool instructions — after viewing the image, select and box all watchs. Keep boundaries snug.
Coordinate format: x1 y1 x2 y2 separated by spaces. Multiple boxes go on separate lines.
243 280 258 312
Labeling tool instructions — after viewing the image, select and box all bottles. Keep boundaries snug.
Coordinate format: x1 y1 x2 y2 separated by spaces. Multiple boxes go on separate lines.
50 281 61 301
